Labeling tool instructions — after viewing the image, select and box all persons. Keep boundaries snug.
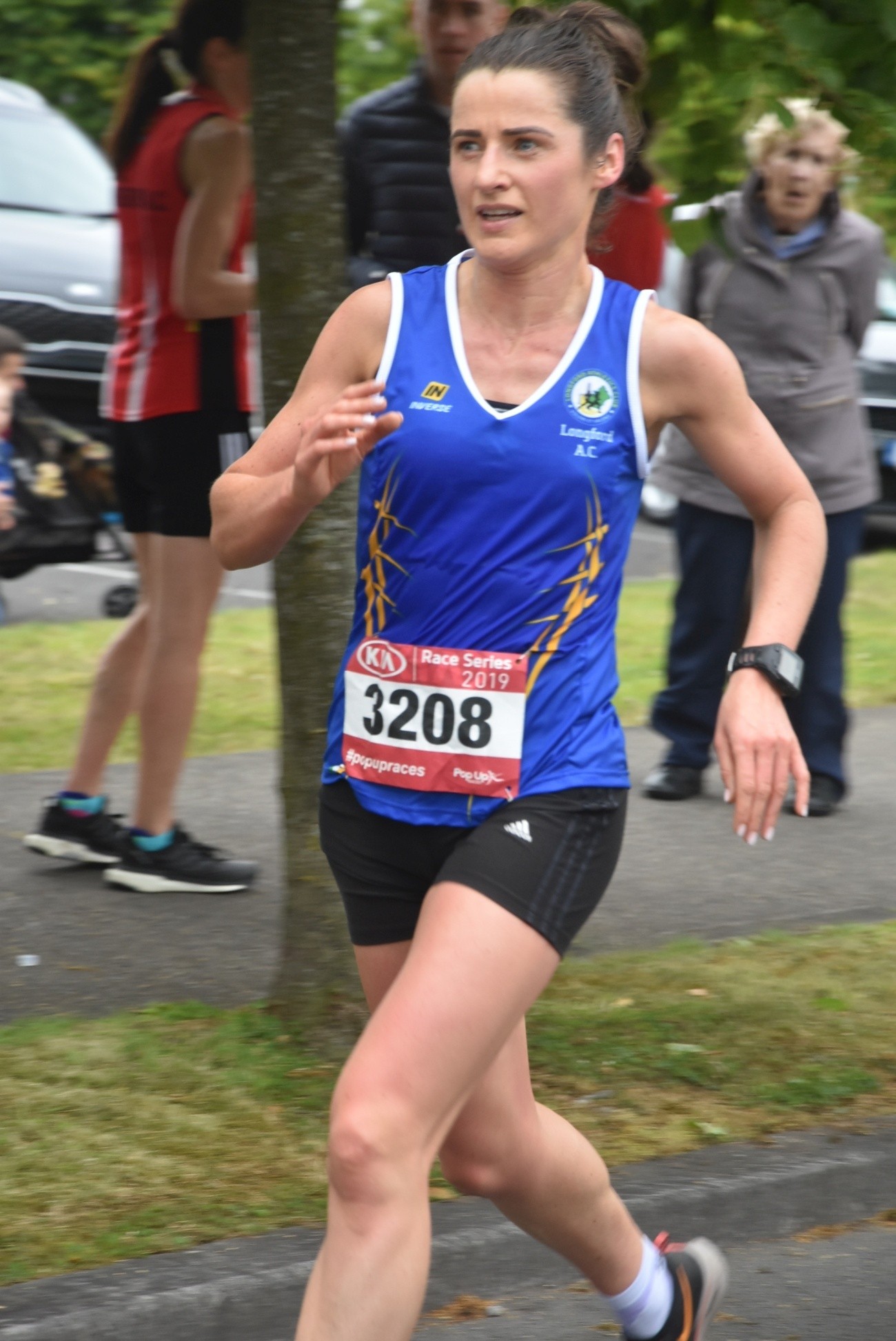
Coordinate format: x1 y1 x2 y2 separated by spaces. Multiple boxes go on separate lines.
208 0 828 1340
1 0 264 895
337 0 673 294
644 95 881 816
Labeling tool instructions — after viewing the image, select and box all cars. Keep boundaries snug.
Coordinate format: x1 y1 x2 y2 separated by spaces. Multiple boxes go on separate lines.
0 76 125 434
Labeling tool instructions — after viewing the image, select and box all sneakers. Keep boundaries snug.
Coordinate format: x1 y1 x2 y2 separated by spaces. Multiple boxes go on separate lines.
24 794 131 864
622 1231 728 1341
103 829 258 893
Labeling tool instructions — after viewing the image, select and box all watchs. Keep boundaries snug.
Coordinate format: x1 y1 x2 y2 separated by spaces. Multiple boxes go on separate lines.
725 641 803 696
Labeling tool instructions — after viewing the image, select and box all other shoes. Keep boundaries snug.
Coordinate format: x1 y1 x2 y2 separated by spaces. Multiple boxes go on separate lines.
783 772 841 816
641 765 702 799
